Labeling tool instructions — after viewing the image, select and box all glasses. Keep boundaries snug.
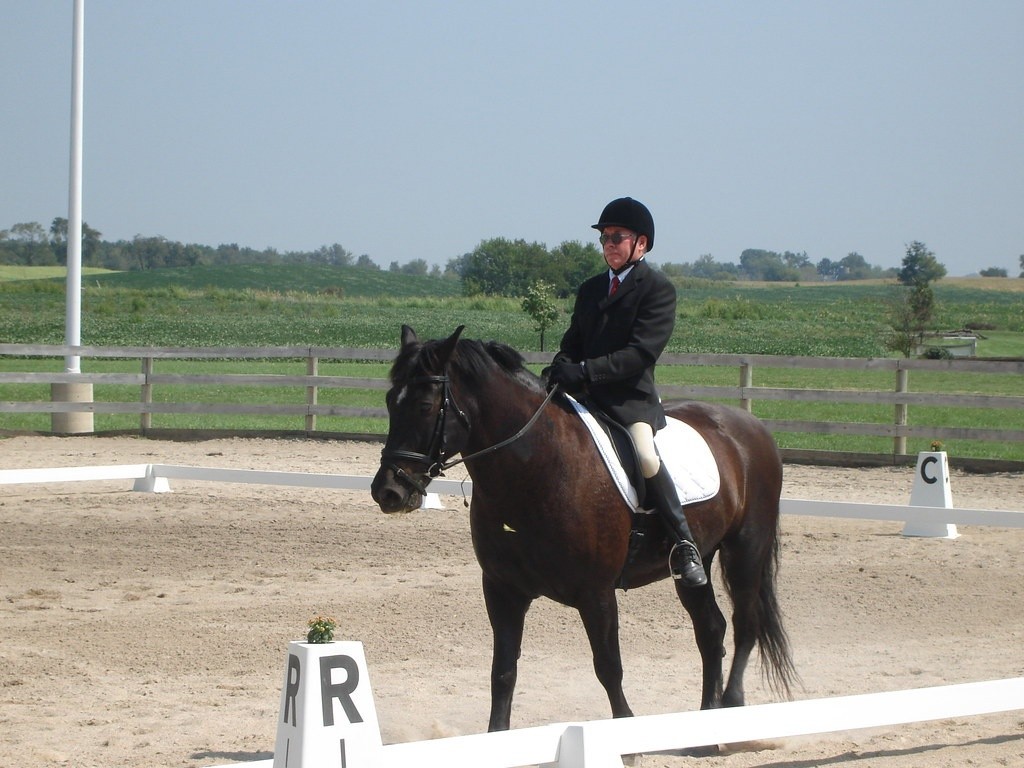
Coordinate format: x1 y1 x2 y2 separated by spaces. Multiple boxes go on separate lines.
599 232 632 245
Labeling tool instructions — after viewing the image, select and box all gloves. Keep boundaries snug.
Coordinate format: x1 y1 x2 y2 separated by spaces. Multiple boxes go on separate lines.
542 362 585 390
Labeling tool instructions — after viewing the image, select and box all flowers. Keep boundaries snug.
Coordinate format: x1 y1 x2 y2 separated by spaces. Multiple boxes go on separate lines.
931 441 945 452
307 616 337 643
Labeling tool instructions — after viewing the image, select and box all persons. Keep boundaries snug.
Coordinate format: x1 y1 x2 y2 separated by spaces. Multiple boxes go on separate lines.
541 197 707 586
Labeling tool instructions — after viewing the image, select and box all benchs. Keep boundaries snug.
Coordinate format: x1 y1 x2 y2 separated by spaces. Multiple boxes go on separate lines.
910 337 976 358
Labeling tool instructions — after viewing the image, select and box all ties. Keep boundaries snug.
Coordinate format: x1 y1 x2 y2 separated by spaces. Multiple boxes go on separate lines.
609 276 619 297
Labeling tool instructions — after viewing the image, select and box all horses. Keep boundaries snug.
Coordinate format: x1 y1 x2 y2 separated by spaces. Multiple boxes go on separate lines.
367 319 812 735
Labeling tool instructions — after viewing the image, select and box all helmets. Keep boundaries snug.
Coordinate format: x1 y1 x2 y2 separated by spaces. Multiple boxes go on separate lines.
592 197 654 252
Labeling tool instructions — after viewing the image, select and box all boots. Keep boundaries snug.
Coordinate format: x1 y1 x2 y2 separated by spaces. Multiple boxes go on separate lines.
644 460 707 587
